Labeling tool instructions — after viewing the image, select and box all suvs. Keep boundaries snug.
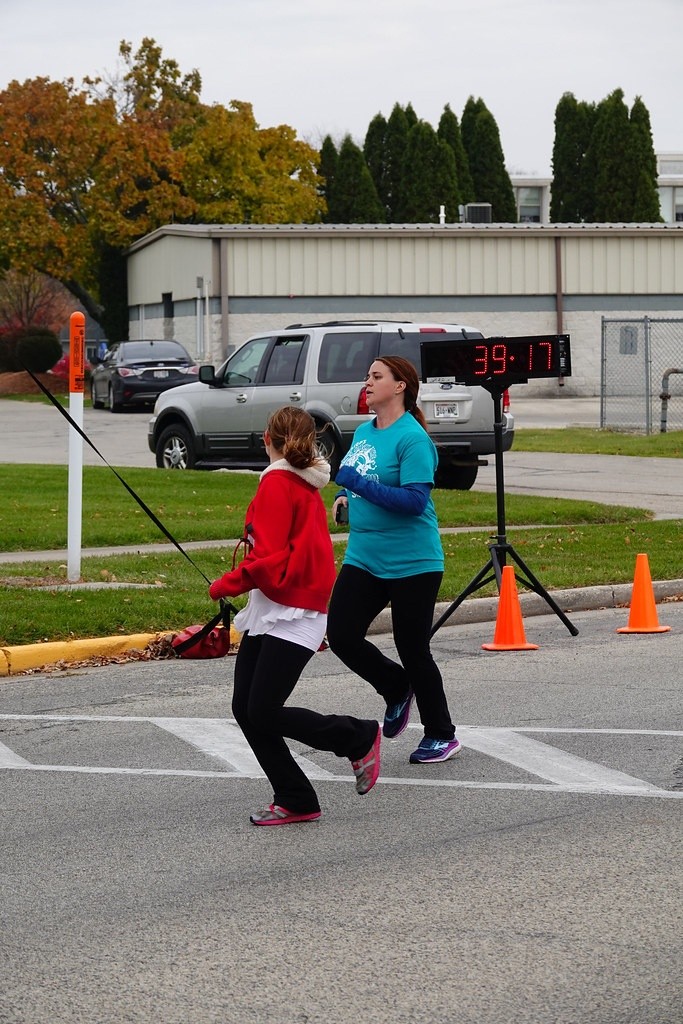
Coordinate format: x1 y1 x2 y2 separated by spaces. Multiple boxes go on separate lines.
148 317 514 490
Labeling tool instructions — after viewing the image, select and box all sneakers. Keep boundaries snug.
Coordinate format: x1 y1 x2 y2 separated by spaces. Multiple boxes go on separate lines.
250 805 321 825
383 683 415 738
409 737 461 763
352 726 381 795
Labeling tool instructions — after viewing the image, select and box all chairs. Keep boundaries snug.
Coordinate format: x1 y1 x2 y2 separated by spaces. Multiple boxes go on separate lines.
277 346 302 382
346 348 365 381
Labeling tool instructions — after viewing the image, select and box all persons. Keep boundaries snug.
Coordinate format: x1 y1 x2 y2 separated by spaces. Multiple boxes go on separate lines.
210 407 381 826
327 356 462 763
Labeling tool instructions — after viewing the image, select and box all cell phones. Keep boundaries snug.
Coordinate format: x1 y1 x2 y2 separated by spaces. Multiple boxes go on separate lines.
336 504 350 524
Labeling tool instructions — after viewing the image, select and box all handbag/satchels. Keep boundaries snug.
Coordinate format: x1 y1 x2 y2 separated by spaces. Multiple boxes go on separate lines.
172 600 229 658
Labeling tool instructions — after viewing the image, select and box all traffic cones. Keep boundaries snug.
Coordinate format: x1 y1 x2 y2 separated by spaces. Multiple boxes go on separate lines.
616 553 671 634
481 565 539 651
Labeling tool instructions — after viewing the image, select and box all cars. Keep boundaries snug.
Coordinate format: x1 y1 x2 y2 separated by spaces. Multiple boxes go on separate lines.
89 339 199 413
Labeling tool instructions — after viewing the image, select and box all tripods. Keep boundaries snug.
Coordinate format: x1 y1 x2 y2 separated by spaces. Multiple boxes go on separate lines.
428 385 581 644
